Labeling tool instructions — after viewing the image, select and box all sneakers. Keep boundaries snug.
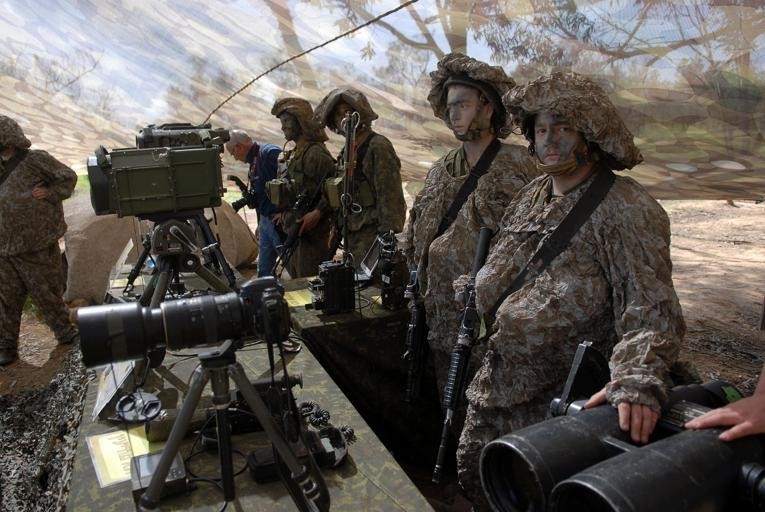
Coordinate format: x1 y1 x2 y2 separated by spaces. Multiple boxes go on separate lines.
0 353 16 365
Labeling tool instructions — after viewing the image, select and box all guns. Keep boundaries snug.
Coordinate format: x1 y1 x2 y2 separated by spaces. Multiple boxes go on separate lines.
124 233 150 293
228 175 260 225
195 213 236 289
400 302 424 402
429 227 494 487
271 176 326 278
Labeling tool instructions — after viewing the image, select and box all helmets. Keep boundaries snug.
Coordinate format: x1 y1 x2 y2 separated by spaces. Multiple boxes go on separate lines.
443 73 505 127
522 115 535 142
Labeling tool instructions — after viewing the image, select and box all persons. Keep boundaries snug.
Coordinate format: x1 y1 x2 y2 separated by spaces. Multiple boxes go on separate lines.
0 116 80 366
226 88 407 286
685 361 765 441
406 83 543 440
452 72 686 511
